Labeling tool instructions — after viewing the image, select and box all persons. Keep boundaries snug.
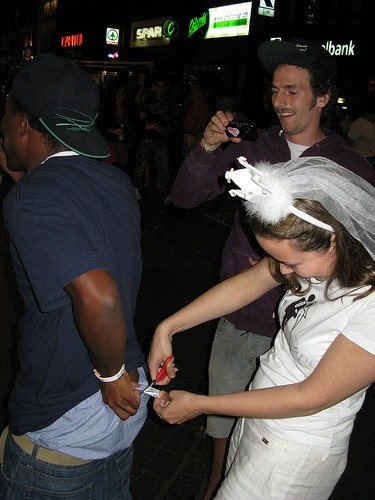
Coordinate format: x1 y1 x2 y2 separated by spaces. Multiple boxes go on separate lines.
333 112 354 138
98 84 131 170
0 52 149 499
347 107 375 165
147 157 375 500
170 41 375 500
183 86 209 157
133 78 168 200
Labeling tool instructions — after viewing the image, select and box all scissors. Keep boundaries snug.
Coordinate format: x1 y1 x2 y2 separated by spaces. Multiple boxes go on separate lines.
137 355 175 401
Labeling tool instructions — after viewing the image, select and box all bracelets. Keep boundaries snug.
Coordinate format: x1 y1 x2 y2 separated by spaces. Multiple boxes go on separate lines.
93 364 126 382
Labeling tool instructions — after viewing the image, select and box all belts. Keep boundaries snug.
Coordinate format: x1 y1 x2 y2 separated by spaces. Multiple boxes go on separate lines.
0 425 89 467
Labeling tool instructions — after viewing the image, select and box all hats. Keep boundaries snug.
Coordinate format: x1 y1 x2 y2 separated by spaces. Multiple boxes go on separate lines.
11 54 112 159
257 38 333 76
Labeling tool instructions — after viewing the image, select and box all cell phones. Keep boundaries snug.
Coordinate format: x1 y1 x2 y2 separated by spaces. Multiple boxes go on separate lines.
227 122 259 141
